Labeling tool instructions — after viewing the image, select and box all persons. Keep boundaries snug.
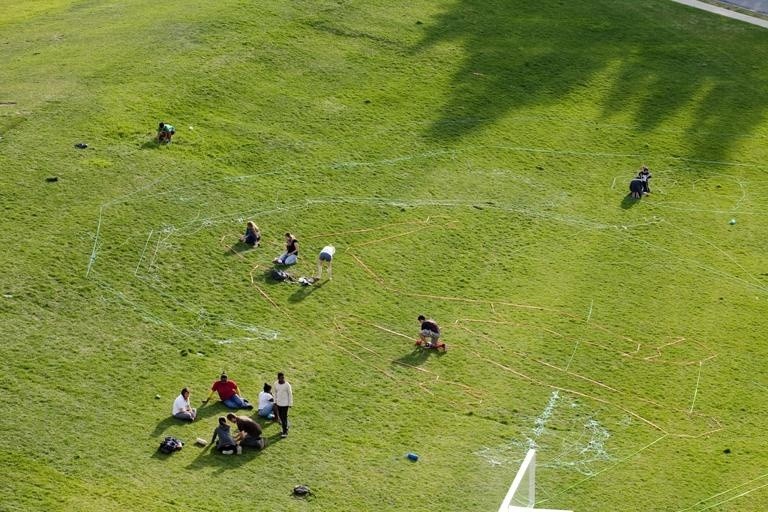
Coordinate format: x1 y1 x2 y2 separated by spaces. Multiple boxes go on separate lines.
629 166 652 198
172 373 294 455
316 243 336 281
417 315 447 352
241 221 261 247
156 122 175 144
272 232 298 265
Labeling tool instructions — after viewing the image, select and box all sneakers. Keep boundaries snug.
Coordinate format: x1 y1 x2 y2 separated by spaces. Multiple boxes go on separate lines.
442 343 447 352
423 342 432 349
282 431 287 438
222 445 242 455
258 436 267 449
